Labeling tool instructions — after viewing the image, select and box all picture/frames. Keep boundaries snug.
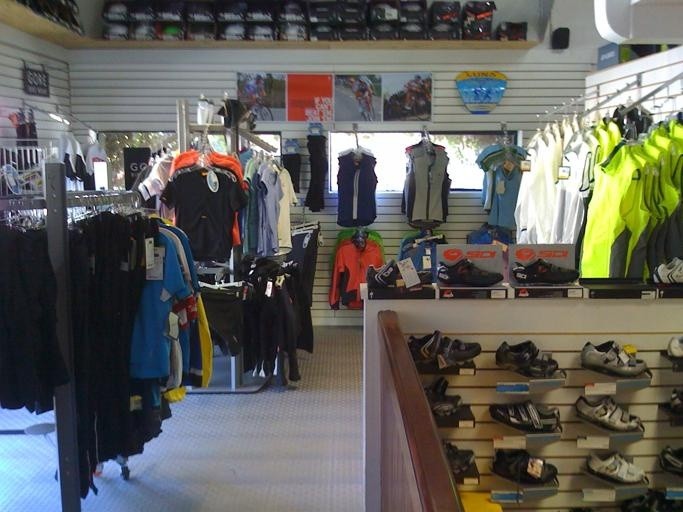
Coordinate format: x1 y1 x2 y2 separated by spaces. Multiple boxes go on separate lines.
236 71 434 124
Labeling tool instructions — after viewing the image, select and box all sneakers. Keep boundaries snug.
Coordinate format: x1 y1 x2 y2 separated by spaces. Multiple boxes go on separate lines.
508 257 580 287
435 257 504 288
488 398 561 434
585 450 647 485
666 335 683 359
652 256 683 284
490 448 559 484
421 377 464 418
620 488 683 512
669 389 683 411
406 329 482 367
366 258 432 291
573 393 641 432
494 340 559 379
580 340 647 377
442 441 475 475
657 444 683 475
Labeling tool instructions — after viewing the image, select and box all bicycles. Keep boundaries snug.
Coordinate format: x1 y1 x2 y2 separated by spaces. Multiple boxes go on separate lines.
385 92 430 121
356 93 376 121
248 96 273 122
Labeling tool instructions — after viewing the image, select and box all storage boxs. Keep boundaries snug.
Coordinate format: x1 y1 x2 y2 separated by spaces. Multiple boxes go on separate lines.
497 22 529 41
101 1 497 41
18 0 83 36
596 42 640 70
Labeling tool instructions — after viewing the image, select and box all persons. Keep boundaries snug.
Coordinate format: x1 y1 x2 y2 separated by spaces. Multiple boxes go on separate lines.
244 74 266 110
386 75 431 114
353 76 375 121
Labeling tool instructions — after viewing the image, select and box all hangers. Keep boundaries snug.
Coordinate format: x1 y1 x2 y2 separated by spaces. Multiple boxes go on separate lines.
352 223 368 250
0 188 155 228
414 227 443 244
245 131 282 174
196 258 257 297
494 121 520 168
337 143 376 168
94 129 104 148
291 220 320 236
531 83 683 181
147 133 178 167
60 117 81 142
481 221 498 238
405 125 450 163
191 131 217 176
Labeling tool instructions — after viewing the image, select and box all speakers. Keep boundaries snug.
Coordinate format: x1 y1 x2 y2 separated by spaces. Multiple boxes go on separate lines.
553 28 569 48
